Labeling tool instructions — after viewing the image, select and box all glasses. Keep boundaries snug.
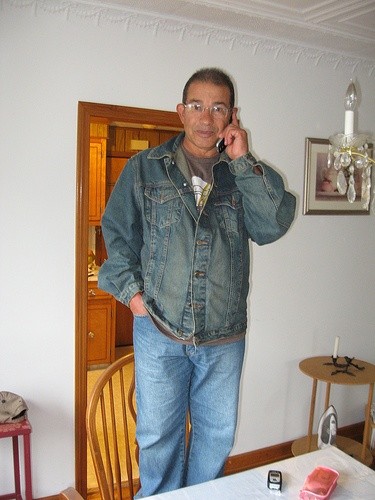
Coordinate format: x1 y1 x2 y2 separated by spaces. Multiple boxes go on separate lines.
186 104 230 119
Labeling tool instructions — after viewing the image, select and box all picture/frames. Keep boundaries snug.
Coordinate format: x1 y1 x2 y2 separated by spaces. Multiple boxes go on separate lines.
303 137 374 216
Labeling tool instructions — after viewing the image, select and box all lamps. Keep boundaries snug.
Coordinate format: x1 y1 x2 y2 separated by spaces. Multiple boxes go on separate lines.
328 81 375 212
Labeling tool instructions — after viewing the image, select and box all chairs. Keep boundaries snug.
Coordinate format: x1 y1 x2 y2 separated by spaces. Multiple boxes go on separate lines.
85 353 192 500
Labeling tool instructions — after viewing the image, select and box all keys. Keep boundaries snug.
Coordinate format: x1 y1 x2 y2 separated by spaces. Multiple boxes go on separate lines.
267 470 282 492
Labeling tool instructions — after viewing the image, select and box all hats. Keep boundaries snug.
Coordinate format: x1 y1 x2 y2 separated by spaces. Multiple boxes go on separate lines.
0 391 29 424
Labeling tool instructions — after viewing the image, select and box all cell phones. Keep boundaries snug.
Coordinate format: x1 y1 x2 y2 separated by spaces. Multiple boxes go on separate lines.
267 470 282 493
216 116 233 153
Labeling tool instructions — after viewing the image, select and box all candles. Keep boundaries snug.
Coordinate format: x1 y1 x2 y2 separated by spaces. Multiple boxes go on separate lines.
332 335 340 360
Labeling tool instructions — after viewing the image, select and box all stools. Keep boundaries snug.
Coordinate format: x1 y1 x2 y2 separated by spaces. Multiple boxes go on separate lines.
0 417 32 500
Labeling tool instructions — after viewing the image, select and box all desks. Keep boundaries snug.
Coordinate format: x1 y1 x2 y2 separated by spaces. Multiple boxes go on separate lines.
139 445 375 500
291 354 375 471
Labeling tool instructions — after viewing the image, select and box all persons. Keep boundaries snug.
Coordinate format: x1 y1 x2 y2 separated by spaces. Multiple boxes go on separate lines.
97 66 301 499
